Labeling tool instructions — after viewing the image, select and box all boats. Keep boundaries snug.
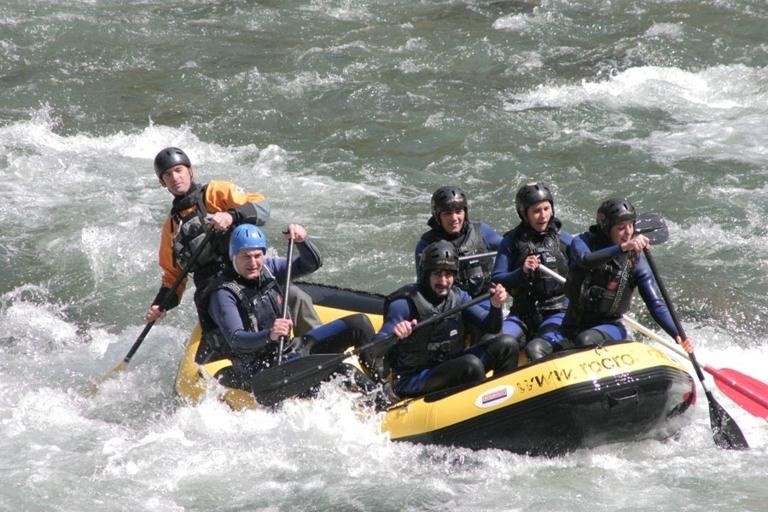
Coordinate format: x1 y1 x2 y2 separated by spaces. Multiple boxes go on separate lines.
174 281 696 458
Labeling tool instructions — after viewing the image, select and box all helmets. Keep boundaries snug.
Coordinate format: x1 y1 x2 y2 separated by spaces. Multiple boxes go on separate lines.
154 147 191 178
419 239 460 286
228 223 267 262
431 186 468 227
515 182 555 224
596 198 636 239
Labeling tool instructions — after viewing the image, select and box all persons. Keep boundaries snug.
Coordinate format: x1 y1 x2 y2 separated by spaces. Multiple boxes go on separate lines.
552 198 694 354
479 181 577 365
370 241 519 400
199 224 384 402
415 186 506 347
143 148 270 366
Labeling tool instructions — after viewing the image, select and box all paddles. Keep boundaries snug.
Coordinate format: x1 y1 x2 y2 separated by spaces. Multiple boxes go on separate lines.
91 225 216 396
458 212 668 264
644 246 749 449
538 263 768 422
252 283 497 406
